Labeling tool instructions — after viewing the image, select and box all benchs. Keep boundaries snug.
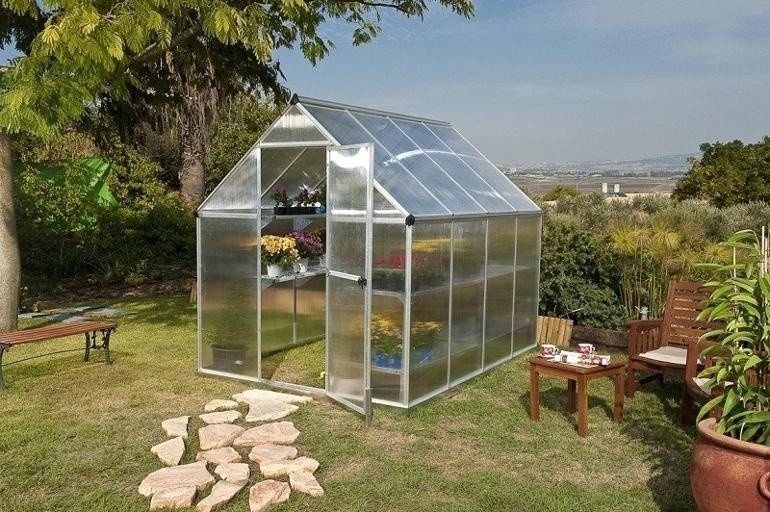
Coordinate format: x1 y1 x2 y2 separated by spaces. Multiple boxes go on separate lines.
0 320 118 388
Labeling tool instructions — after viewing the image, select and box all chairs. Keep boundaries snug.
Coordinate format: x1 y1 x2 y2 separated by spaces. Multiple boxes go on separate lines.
626 280 740 425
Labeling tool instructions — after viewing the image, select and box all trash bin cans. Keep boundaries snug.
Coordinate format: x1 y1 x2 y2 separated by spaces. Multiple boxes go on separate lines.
602 183 607 193
614 184 620 192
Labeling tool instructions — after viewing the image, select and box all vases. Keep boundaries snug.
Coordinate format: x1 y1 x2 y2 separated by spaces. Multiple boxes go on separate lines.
376 347 434 369
372 278 404 292
265 255 326 278
273 203 320 214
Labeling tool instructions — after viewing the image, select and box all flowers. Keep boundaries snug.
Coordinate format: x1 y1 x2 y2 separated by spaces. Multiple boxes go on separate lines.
371 312 444 352
372 253 447 280
261 229 326 262
270 186 326 203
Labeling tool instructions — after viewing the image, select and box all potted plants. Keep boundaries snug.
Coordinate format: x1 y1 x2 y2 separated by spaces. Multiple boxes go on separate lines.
211 275 255 375
691 228 770 512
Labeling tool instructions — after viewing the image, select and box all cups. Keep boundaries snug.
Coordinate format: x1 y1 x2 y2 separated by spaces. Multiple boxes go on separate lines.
540 343 559 356
577 343 596 354
